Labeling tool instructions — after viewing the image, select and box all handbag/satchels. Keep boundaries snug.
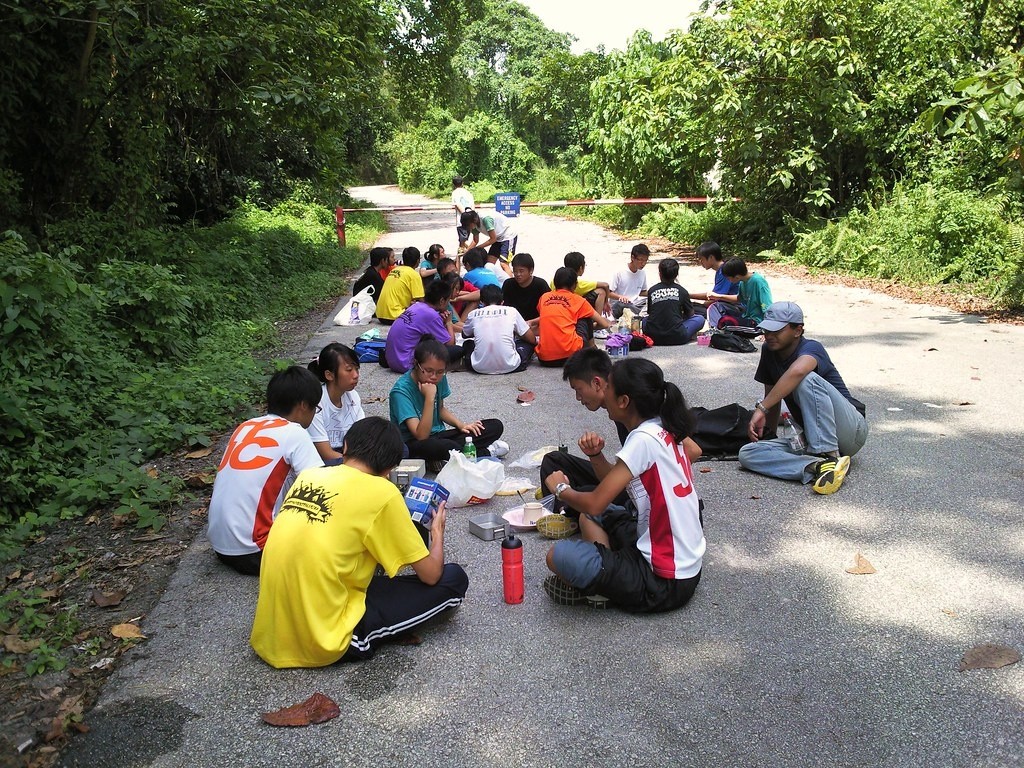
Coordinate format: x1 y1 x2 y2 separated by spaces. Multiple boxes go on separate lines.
435 449 506 509
353 335 387 363
708 333 757 352
333 284 376 326
686 402 778 456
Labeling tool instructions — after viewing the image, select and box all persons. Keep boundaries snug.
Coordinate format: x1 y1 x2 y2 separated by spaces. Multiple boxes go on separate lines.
388 334 509 474
534 347 707 615
352 175 774 377
738 300 869 495
207 365 327 576
307 341 410 469
248 415 470 670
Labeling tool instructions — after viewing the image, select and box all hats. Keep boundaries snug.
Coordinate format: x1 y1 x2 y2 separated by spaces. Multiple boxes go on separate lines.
756 300 804 332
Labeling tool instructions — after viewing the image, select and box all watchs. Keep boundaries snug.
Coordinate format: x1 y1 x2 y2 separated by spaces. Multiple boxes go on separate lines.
555 483 571 501
754 399 770 415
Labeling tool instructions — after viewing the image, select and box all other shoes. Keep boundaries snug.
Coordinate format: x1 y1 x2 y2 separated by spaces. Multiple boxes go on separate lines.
536 514 579 539
544 575 612 609
536 487 543 499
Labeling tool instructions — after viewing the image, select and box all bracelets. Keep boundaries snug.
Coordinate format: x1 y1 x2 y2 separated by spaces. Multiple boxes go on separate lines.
587 451 601 457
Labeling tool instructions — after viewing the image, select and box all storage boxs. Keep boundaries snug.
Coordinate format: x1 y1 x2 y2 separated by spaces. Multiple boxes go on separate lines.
405 476 451 532
697 336 711 345
608 345 629 355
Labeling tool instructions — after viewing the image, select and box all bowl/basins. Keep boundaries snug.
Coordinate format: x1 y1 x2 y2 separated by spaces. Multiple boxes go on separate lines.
469 512 510 541
501 507 553 530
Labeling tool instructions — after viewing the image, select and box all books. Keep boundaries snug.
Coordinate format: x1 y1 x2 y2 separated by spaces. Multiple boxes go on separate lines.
404 476 450 531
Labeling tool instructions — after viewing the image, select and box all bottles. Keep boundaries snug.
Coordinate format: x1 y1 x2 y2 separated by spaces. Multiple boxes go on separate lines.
782 412 808 456
617 316 627 335
501 534 524 605
463 437 477 464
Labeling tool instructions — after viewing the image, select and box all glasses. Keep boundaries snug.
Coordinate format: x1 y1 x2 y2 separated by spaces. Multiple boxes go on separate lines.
635 257 649 264
305 400 322 414
761 328 783 334
418 363 447 376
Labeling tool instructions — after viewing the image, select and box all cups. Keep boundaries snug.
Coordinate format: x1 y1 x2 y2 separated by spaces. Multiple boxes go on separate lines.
524 503 542 526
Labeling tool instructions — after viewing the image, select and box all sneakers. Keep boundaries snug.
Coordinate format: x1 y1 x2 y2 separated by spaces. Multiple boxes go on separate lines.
458 246 466 254
487 440 510 458
428 459 448 475
812 456 851 494
